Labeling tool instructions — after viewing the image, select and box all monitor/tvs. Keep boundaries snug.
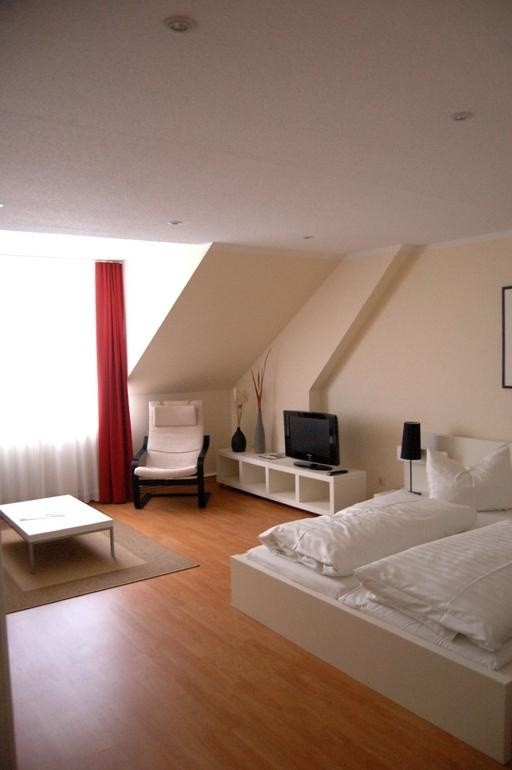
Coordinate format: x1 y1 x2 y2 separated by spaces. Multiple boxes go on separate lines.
283 410 340 466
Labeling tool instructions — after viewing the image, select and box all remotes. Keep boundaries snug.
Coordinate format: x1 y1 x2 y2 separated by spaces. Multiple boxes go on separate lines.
327 470 348 476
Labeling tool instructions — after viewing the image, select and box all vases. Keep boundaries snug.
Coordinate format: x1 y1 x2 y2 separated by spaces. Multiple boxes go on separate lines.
230 411 265 453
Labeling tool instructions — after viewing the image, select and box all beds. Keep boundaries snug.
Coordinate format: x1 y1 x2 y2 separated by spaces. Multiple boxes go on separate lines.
229 430 511 767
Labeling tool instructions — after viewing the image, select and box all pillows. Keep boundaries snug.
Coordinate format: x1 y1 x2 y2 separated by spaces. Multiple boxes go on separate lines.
424 443 512 512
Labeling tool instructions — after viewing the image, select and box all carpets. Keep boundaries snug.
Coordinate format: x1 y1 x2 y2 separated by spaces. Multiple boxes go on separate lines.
1 515 200 617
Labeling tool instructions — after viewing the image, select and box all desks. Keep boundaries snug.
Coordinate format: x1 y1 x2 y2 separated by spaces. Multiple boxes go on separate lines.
0 492 117 573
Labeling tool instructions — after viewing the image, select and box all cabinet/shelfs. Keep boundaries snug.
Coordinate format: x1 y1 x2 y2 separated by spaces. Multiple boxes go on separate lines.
215 445 368 517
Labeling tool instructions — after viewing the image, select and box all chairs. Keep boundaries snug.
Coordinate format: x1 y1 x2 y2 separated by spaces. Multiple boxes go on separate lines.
127 398 212 512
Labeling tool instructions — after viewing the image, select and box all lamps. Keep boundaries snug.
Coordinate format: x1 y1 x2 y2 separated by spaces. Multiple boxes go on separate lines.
397 419 422 496
